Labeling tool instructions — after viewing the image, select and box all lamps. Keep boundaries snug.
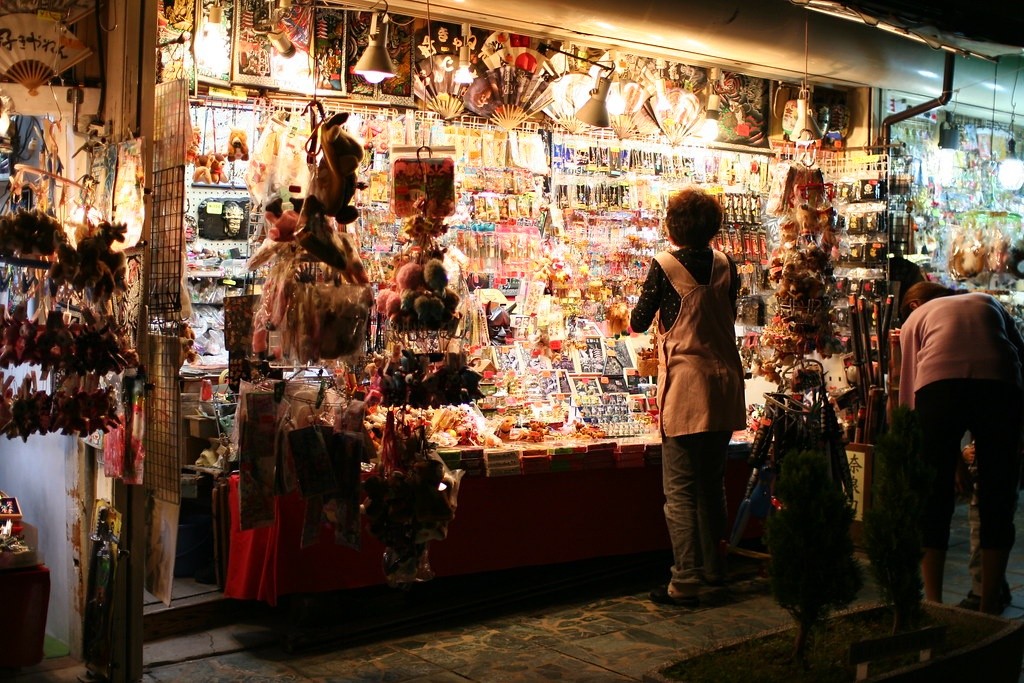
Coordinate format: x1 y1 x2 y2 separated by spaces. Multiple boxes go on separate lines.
190 5 827 144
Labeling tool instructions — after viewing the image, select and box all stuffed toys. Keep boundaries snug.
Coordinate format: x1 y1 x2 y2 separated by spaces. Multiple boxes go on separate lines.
751 166 843 384
0 111 543 584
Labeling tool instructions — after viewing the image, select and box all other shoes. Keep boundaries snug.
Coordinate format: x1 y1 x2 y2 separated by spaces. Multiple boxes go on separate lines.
648 587 699 608
956 592 982 610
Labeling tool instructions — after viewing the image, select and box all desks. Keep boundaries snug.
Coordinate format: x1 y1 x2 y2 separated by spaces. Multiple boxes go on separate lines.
224 430 873 648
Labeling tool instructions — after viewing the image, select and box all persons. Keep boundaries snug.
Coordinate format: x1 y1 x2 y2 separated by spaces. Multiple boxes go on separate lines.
897 282 1024 614
630 189 747 607
